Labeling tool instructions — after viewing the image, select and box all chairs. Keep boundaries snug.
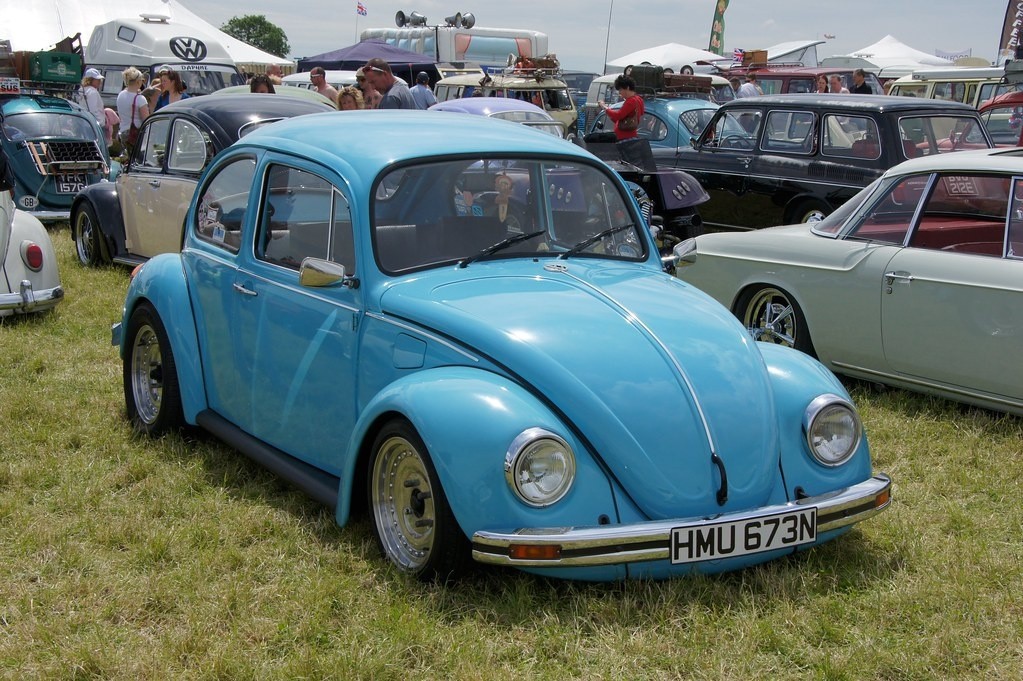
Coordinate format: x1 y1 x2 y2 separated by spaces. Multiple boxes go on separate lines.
291 221 356 277
438 216 508 260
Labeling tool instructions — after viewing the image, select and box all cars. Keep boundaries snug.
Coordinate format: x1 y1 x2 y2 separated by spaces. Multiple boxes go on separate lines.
110 110 893 581
68 92 403 268
649 92 1000 229
673 147 1023 417
0 93 125 224
384 96 712 227
0 149 66 317
918 89 1023 189
586 96 754 149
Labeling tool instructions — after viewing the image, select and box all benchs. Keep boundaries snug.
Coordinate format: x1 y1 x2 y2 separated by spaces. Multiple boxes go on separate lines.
224 224 417 276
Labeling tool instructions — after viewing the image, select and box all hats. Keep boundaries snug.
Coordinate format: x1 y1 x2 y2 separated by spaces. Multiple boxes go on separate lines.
417 71 429 83
86 68 105 80
157 64 171 73
356 67 366 77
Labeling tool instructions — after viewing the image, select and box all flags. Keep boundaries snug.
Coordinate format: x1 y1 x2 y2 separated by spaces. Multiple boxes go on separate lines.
357 2 368 16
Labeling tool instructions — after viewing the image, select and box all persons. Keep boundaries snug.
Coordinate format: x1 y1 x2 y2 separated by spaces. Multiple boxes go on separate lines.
76 63 189 169
598 74 644 139
727 70 764 100
816 68 895 95
247 58 440 111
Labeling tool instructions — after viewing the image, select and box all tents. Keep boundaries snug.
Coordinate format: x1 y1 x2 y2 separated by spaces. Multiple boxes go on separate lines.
298 35 445 93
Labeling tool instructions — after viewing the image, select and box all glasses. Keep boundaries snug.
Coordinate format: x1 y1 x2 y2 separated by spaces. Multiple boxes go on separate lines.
745 79 755 83
362 65 385 73
310 74 321 78
355 76 366 82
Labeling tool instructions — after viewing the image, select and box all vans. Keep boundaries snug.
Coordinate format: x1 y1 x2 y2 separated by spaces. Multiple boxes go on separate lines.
280 70 410 92
425 65 610 134
715 66 892 137
884 60 1023 132
583 73 625 128
154 84 337 150
80 11 248 113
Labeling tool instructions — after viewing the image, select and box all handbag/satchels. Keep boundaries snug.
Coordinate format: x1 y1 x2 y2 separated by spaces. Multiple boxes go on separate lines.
618 97 637 132
128 94 141 146
